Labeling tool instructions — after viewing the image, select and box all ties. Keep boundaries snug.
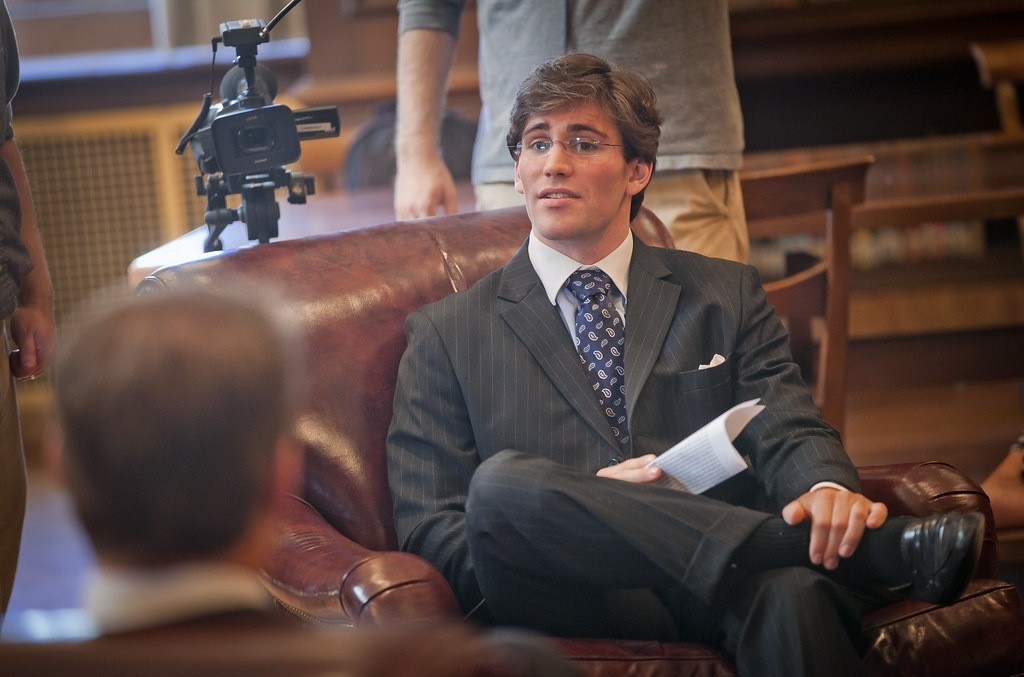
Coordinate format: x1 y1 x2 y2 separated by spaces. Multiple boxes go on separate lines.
566 266 632 458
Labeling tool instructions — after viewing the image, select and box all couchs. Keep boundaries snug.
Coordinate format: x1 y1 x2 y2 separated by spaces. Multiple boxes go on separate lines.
137 197 1024 677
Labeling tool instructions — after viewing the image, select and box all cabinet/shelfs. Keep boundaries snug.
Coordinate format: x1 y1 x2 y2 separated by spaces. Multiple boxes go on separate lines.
736 127 1024 464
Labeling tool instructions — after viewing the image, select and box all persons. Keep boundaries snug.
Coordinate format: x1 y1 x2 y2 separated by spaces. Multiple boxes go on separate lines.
0 0 56 623
42 273 305 641
393 0 751 264
386 53 986 677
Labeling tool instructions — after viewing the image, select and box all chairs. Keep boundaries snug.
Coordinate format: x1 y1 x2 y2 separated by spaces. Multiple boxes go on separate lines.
732 156 874 455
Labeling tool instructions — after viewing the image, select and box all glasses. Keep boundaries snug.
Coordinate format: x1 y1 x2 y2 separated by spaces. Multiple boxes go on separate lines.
515 133 629 159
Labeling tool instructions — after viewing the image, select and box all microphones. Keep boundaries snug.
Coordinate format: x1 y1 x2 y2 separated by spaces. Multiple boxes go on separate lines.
221 62 278 105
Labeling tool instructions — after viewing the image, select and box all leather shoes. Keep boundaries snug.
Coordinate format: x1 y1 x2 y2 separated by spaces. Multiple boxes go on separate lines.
894 506 985 602
